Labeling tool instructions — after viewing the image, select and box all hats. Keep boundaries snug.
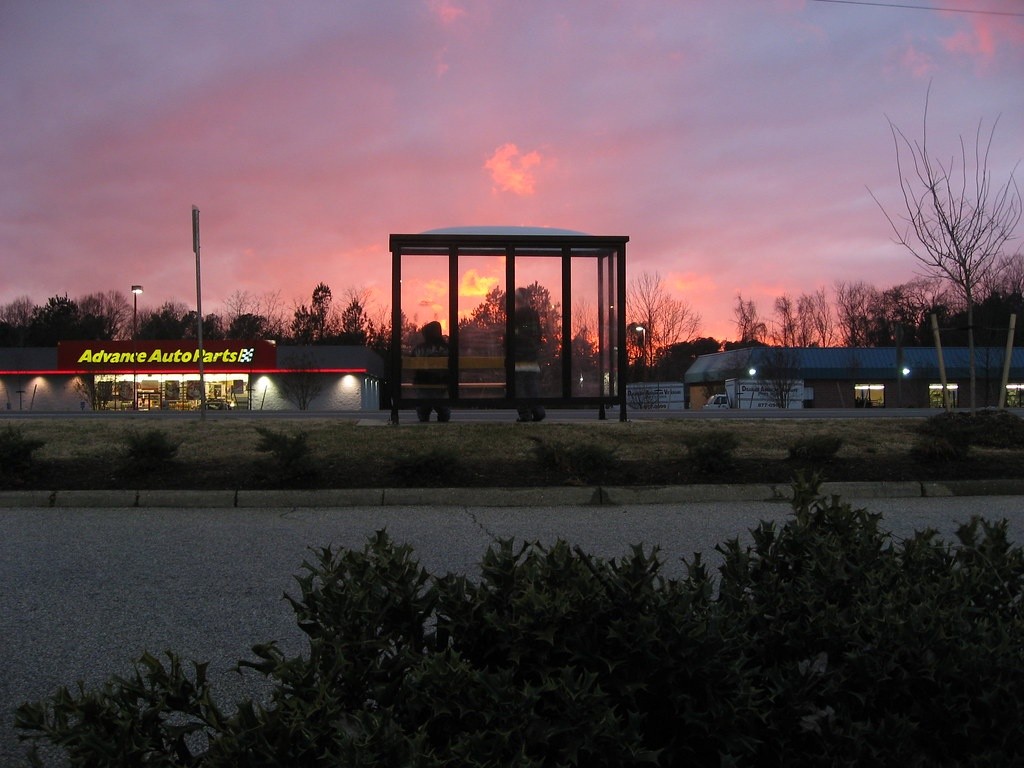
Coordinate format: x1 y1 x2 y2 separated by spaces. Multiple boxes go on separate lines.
421 321 443 342
517 288 532 301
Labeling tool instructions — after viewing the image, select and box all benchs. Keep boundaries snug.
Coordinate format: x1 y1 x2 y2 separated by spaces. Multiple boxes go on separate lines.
205 401 231 410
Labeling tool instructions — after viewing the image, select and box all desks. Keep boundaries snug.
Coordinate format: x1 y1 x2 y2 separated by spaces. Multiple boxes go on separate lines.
208 397 225 402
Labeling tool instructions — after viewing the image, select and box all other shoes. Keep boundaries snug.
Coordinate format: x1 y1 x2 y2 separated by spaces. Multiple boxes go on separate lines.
439 415 450 422
517 416 531 422
418 414 429 422
532 411 546 422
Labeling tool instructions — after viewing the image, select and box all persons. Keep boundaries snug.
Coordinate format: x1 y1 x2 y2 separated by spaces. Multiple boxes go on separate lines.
412 320 452 422
509 288 547 422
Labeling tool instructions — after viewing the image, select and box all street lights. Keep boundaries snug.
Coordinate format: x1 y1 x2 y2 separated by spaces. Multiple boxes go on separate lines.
635 326 646 383
130 284 144 410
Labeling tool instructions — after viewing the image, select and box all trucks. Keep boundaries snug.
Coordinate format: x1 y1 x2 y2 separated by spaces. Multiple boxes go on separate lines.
702 378 805 410
608 382 685 411
